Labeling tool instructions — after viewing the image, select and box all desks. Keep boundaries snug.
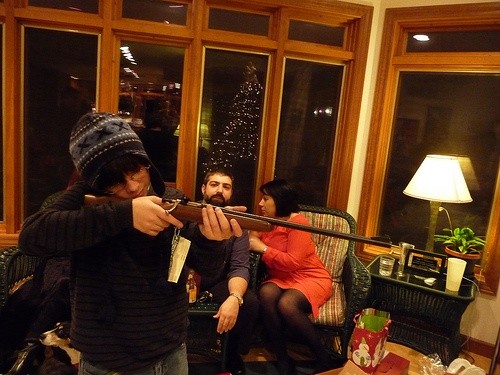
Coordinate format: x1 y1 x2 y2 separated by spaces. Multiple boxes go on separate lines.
366 257 475 365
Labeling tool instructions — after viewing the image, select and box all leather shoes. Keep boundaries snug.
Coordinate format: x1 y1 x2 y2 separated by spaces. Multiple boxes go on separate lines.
10 344 39 373
227 356 245 375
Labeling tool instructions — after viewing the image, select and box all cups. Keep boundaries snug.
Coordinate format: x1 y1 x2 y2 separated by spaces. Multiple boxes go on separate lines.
378 255 395 276
445 258 467 292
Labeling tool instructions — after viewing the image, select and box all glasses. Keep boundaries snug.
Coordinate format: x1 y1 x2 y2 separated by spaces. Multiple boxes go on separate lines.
104 163 150 194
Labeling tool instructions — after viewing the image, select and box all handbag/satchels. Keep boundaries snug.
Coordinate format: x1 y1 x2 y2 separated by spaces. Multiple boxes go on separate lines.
351 307 390 373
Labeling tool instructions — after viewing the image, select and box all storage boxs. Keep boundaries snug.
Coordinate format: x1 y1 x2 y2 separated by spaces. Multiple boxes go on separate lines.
373 353 410 375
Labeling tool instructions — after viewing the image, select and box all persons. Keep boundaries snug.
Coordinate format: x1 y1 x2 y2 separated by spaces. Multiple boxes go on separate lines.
0 248 72 374
249 179 333 375
173 166 263 375
18 112 247 375
135 102 178 176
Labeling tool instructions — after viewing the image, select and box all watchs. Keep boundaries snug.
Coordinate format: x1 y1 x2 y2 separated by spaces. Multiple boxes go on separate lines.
229 293 243 306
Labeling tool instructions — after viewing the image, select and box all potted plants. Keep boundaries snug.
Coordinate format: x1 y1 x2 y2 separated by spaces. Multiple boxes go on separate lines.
433 206 486 275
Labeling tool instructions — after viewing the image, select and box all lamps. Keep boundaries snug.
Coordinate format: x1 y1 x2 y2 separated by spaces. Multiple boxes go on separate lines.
403 154 473 252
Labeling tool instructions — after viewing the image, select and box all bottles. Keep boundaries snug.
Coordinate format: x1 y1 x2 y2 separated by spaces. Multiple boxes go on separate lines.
184 269 197 307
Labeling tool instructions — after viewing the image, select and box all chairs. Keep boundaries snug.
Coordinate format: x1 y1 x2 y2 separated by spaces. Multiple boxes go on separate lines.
185 204 371 375
0 191 66 307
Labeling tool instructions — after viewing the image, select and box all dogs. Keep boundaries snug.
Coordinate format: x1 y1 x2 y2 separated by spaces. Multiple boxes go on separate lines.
37 320 81 369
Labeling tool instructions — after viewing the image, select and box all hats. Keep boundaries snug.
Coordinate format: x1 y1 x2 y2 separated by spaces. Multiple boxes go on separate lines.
69 111 165 196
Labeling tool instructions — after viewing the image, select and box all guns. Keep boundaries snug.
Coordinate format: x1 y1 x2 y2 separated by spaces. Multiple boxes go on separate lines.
81 193 393 250
